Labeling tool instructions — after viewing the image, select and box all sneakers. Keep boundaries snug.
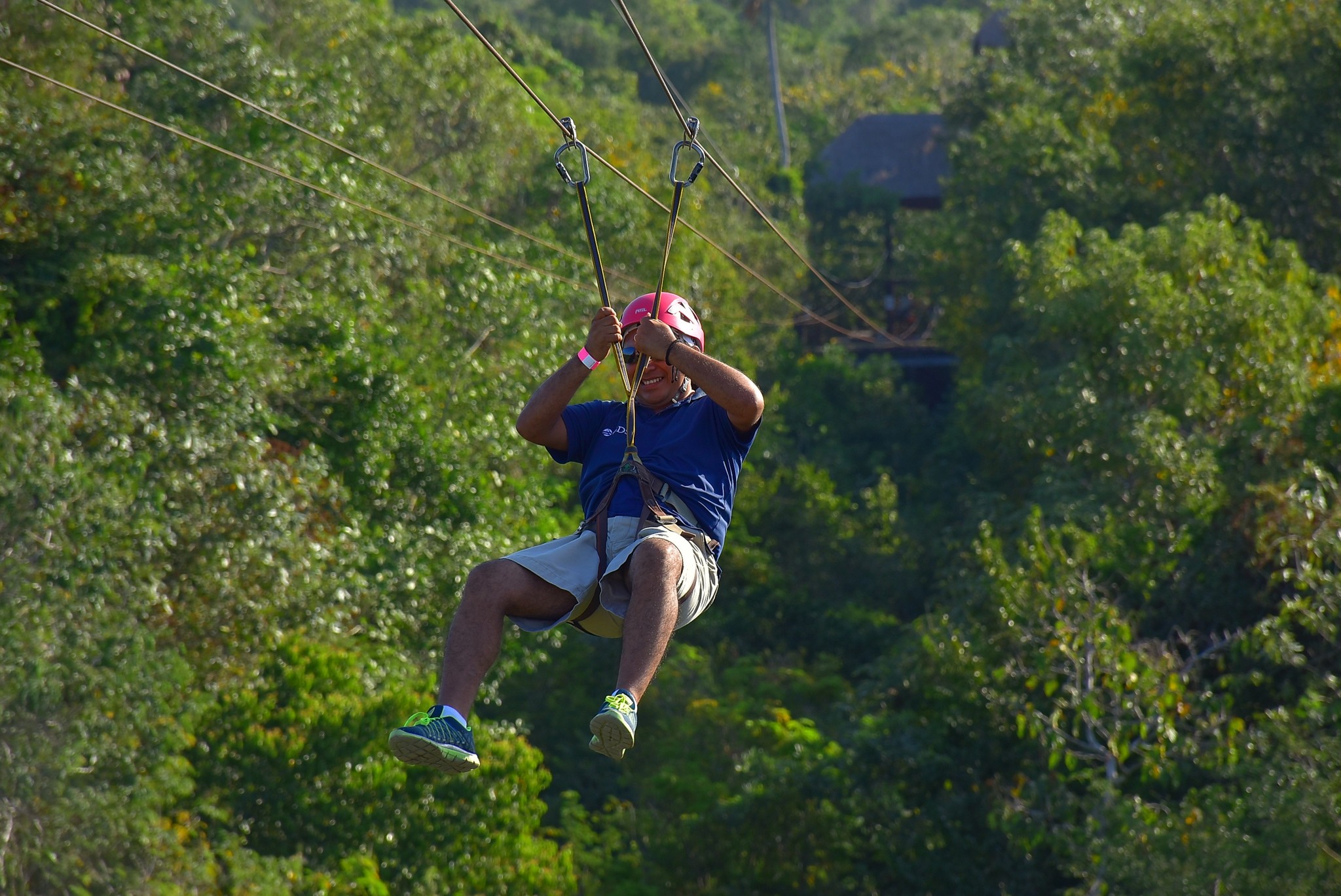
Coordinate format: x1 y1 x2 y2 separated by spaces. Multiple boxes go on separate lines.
388 705 480 774
588 688 638 759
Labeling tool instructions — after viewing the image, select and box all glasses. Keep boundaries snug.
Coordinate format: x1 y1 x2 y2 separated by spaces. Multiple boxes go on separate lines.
622 346 640 364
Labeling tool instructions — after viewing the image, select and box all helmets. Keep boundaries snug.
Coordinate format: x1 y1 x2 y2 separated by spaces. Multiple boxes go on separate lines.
619 292 704 354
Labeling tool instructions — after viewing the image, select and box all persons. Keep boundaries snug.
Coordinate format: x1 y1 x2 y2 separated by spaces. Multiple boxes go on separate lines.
388 290 766 773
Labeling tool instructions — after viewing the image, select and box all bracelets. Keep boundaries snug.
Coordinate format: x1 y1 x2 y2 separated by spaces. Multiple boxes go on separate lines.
665 339 683 367
577 345 603 370
